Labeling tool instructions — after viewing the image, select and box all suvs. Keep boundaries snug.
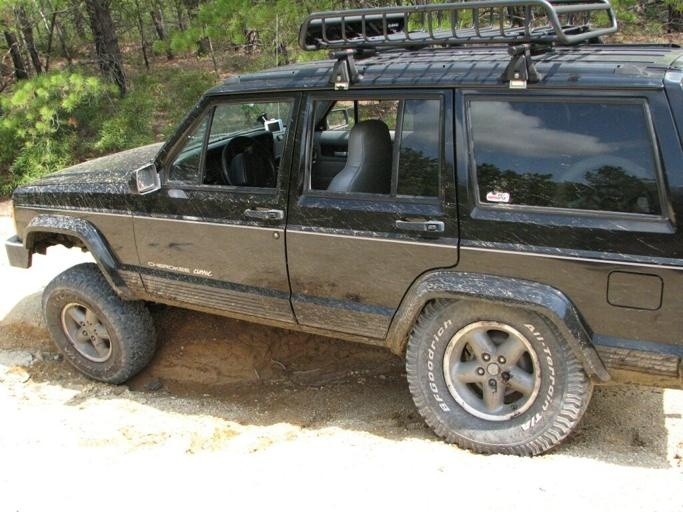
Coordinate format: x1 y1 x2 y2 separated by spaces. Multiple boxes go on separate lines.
5 0 682 460
